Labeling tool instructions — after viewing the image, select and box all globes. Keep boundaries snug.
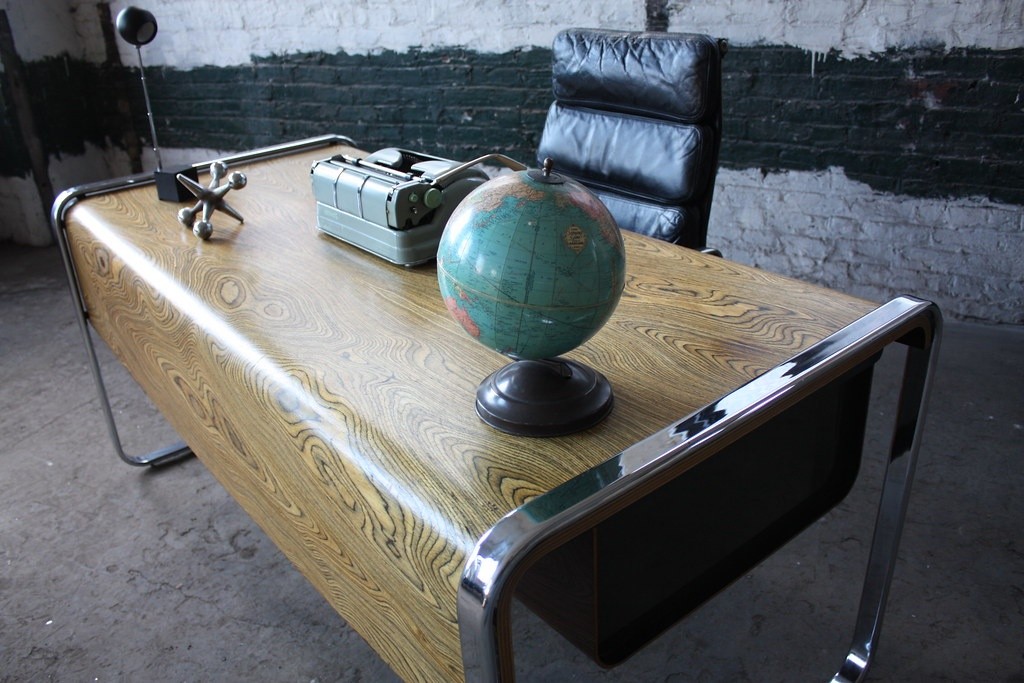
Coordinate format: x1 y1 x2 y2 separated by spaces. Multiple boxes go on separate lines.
434 154 628 439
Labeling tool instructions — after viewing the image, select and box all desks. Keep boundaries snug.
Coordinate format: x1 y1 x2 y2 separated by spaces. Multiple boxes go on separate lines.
48 132 945 683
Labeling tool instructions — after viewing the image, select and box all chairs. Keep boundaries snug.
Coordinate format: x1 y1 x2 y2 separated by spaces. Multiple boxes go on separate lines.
538 26 729 256
